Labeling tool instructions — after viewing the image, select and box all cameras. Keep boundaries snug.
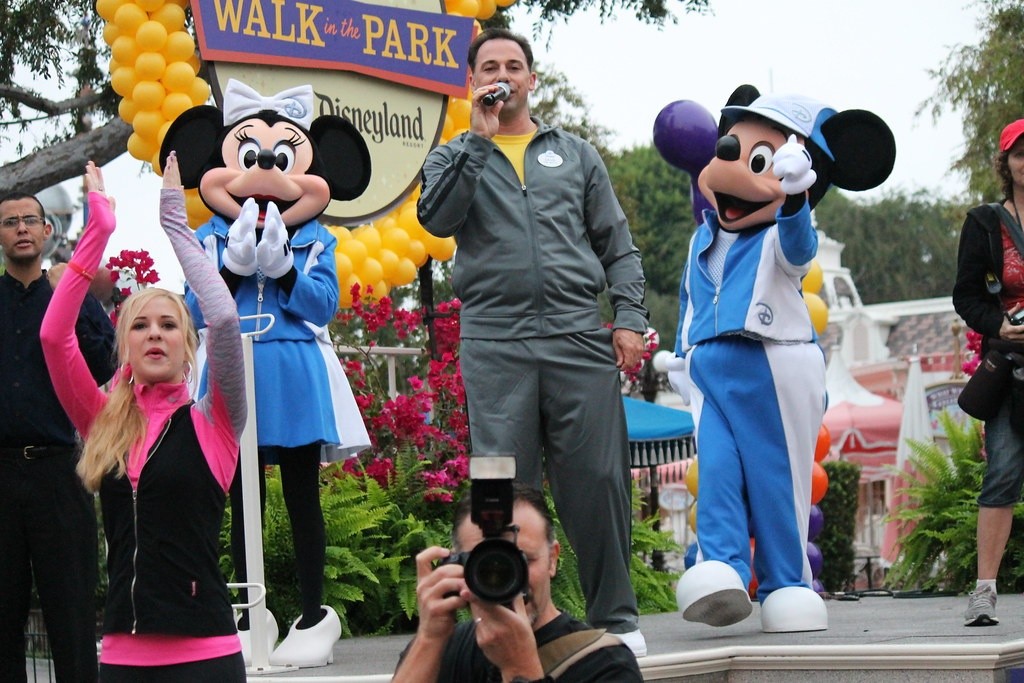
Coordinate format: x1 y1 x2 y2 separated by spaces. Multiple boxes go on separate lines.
437 449 530 612
1009 308 1024 326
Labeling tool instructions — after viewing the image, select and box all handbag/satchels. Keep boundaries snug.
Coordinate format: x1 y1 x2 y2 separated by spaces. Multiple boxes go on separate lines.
957 350 1010 422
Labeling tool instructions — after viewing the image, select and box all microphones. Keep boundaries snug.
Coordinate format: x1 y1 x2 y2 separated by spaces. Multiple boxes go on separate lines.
482 82 511 107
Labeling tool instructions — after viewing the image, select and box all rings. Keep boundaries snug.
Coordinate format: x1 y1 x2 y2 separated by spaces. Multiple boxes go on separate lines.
637 360 640 362
98 187 103 191
475 618 483 623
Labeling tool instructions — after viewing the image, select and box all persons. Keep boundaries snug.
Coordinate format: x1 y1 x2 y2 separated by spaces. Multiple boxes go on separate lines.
0 188 120 683
390 479 644 683
40 151 248 683
952 119 1024 627
417 30 650 659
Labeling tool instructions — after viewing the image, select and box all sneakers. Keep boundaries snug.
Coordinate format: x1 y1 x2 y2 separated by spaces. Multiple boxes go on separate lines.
612 626 647 658
963 582 998 625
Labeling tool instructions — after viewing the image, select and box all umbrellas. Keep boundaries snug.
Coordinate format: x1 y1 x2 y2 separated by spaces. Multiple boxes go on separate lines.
882 343 934 560
823 342 904 541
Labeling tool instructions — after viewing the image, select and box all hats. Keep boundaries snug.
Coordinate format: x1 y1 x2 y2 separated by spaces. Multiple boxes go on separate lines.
999 118 1024 153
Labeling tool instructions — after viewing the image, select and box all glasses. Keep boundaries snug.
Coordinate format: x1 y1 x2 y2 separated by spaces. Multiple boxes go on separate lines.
0 217 47 232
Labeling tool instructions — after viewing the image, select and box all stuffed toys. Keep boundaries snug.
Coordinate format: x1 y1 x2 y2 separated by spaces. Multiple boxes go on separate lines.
668 84 896 631
159 79 371 665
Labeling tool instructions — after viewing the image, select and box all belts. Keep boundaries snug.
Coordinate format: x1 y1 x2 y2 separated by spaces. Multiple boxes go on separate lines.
0 442 80 460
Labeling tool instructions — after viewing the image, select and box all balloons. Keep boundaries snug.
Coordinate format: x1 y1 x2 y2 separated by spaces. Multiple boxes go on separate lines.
185 187 214 230
653 100 718 175
806 505 824 592
444 0 516 37
684 460 759 597
96 0 210 176
802 260 828 336
324 183 456 308
438 86 475 146
811 425 831 504
